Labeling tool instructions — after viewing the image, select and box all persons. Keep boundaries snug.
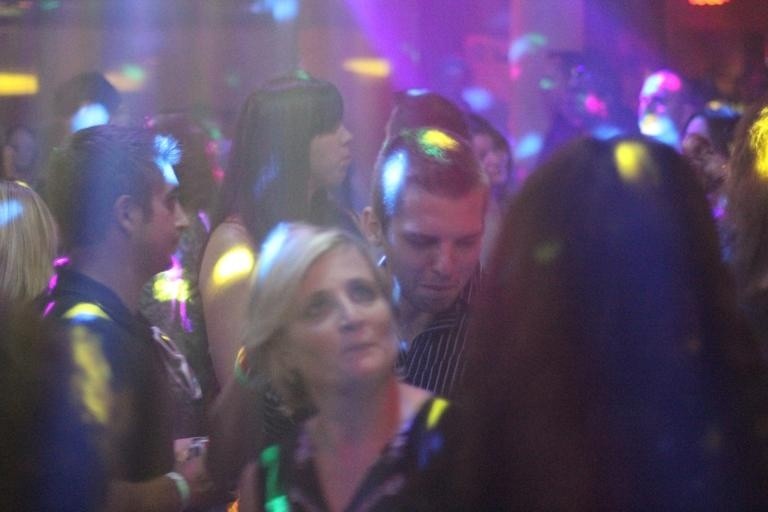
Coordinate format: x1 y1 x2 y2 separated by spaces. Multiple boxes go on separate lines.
1 52 766 512
